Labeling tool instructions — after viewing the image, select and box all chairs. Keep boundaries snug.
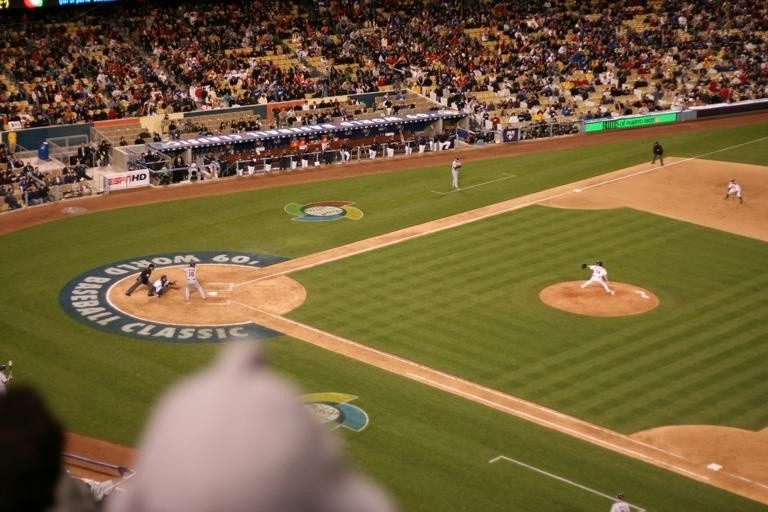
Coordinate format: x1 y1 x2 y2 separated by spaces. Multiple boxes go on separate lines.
98 93 443 150
